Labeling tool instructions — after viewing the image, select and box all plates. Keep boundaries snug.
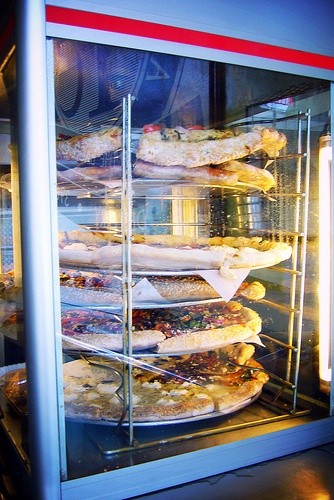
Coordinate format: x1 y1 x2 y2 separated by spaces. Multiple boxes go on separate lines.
57 177 251 198
61 295 238 311
62 345 190 356
65 359 260 425
60 259 275 275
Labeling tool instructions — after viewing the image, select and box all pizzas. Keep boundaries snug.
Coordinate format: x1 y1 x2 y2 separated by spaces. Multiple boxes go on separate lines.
0 122 289 425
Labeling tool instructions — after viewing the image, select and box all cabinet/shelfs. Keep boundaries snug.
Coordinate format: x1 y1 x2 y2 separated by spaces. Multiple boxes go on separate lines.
1 1 333 500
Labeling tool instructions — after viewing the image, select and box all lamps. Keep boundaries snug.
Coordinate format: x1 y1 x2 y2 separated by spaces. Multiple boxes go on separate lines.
315 133 332 396
